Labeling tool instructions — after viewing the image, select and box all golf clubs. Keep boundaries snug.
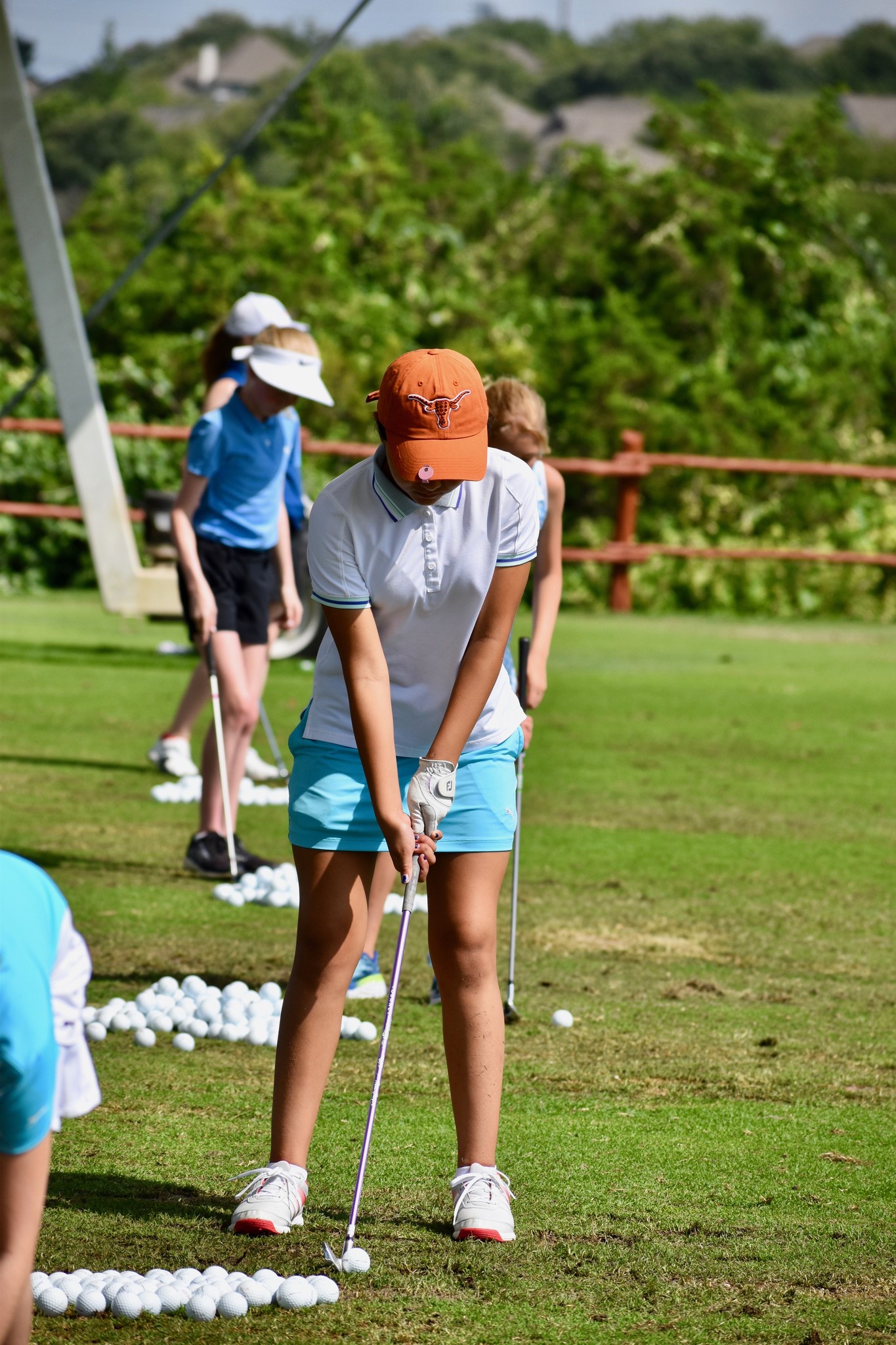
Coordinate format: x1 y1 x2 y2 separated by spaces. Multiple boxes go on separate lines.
259 700 290 781
321 829 427 1276
501 747 527 1025
201 632 245 886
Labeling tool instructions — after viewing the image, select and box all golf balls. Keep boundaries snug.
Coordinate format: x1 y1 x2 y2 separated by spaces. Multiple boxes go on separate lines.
210 861 428 915
149 773 289 806
341 1247 371 1275
30 1265 340 1323
81 975 379 1052
551 1010 574 1028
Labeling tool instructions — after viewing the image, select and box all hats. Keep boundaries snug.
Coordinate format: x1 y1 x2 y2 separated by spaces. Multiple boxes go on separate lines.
231 332 339 407
365 347 488 484
226 290 308 338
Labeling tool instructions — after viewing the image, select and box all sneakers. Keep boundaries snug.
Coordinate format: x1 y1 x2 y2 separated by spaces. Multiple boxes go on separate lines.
449 1162 517 1243
347 951 386 1000
226 1161 310 1235
426 953 443 1004
183 827 277 880
146 730 199 777
245 746 279 782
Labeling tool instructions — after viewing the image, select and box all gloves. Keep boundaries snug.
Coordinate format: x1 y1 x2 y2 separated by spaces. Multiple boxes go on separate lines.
406 756 458 842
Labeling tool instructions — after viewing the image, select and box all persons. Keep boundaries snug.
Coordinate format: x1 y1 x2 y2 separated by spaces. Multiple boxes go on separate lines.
233 348 543 1244
0 851 100 1345
150 291 565 1009
